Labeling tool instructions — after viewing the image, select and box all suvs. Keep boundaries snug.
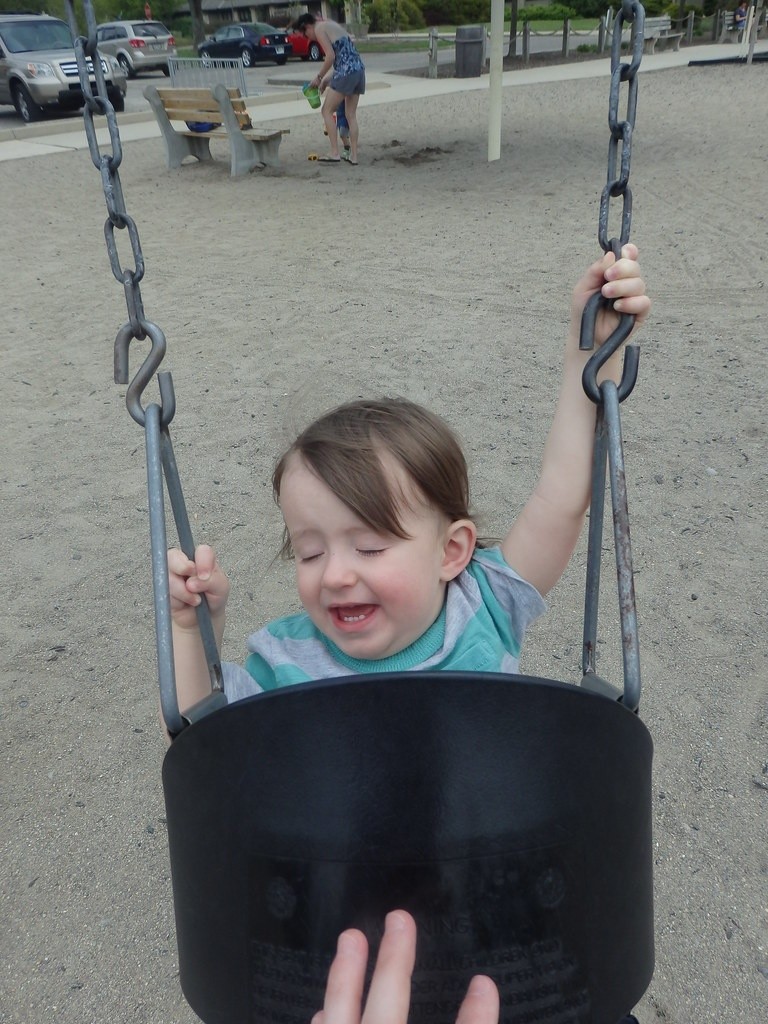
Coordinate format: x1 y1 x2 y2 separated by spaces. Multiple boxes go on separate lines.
97 20 178 80
0 10 127 122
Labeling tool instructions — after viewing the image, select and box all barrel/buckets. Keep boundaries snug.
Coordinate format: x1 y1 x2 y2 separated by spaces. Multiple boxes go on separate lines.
304 86 321 109
453 24 484 78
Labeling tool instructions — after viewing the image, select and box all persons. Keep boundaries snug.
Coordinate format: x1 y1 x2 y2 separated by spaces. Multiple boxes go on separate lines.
735 0 747 43
161 242 652 747
291 11 366 165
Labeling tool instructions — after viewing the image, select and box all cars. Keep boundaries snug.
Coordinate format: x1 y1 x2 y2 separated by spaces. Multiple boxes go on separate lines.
198 22 293 69
287 22 324 62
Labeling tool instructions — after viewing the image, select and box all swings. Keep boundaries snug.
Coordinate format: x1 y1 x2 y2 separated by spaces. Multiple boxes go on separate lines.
62 2 654 1024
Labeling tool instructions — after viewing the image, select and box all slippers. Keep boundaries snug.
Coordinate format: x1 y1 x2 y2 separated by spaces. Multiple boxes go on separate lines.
317 153 341 162
344 154 358 165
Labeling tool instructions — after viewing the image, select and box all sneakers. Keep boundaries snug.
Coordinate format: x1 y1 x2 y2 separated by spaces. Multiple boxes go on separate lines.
341 147 351 159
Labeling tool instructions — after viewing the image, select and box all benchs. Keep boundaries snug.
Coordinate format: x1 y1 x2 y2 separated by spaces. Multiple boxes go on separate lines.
142 82 291 177
627 15 685 54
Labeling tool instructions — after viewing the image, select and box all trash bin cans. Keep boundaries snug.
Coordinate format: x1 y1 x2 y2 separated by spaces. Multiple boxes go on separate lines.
455 24 485 78
